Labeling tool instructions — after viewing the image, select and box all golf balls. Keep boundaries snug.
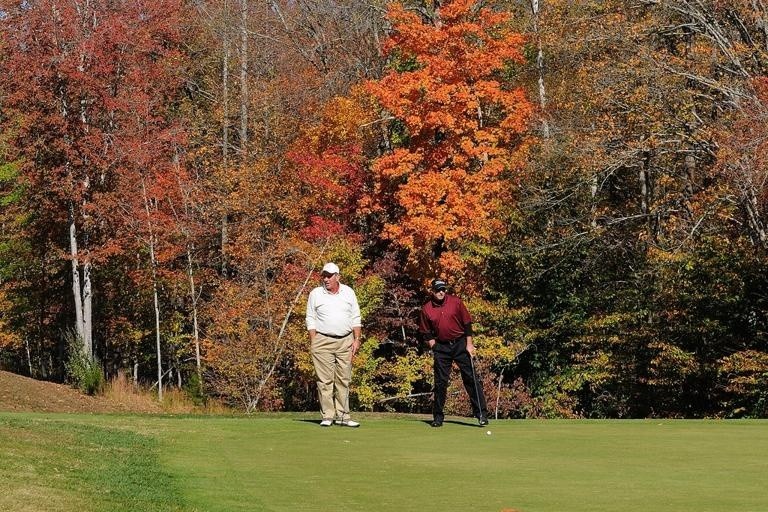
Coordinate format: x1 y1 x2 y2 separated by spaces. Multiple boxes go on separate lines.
486 430 492 436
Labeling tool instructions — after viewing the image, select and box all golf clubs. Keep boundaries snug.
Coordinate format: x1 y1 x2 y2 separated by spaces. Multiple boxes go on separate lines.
341 354 354 426
470 353 487 425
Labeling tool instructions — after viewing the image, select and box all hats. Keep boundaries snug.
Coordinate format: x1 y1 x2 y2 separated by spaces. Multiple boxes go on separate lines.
431 279 446 291
320 262 339 276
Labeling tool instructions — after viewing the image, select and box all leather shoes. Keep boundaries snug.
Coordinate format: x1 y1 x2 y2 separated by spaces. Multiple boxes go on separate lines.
475 410 489 426
430 420 442 427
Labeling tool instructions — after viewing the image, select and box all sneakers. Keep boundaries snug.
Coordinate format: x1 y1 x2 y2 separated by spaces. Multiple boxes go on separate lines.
319 420 333 426
334 419 360 427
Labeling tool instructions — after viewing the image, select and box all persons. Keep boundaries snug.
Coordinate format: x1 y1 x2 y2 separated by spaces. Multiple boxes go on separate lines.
418 279 489 426
306 262 362 427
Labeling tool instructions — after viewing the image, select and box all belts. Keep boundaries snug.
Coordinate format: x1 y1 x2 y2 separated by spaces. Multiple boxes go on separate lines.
316 331 352 339
436 338 459 346
